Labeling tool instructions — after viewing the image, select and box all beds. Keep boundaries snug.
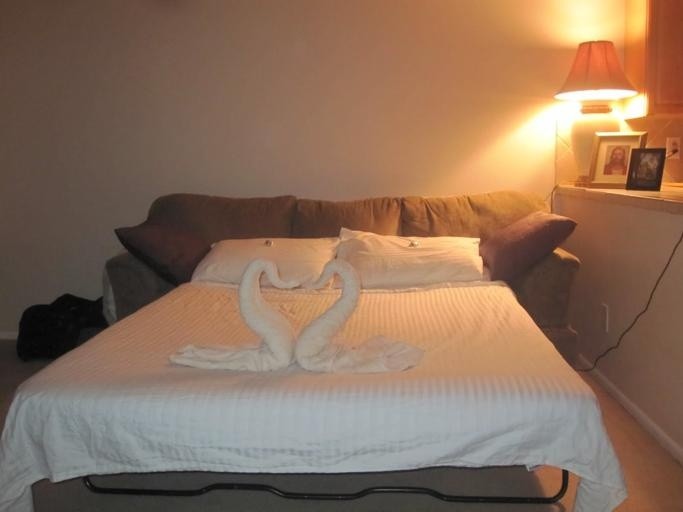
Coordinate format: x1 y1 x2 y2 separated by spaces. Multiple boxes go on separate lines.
5 232 600 511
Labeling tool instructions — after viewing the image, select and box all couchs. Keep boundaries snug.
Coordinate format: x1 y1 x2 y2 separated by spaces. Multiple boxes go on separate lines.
100 246 582 353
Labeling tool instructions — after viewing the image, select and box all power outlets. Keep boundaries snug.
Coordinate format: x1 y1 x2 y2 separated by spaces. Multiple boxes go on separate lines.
666 136 680 159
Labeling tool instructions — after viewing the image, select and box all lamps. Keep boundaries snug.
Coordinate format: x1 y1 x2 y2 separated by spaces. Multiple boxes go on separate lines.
553 38 639 191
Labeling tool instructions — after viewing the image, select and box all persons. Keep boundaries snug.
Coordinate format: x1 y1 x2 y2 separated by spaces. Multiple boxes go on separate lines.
604 146 627 175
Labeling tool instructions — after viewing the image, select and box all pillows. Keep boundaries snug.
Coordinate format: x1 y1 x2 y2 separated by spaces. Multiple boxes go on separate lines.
191 237 337 288
113 215 219 286
479 211 579 281
338 227 483 288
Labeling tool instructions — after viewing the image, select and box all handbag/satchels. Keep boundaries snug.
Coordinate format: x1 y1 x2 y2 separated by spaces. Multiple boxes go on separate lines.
15 292 109 363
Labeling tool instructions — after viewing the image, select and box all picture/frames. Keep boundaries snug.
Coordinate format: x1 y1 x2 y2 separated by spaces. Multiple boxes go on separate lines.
586 130 649 188
625 147 666 191
